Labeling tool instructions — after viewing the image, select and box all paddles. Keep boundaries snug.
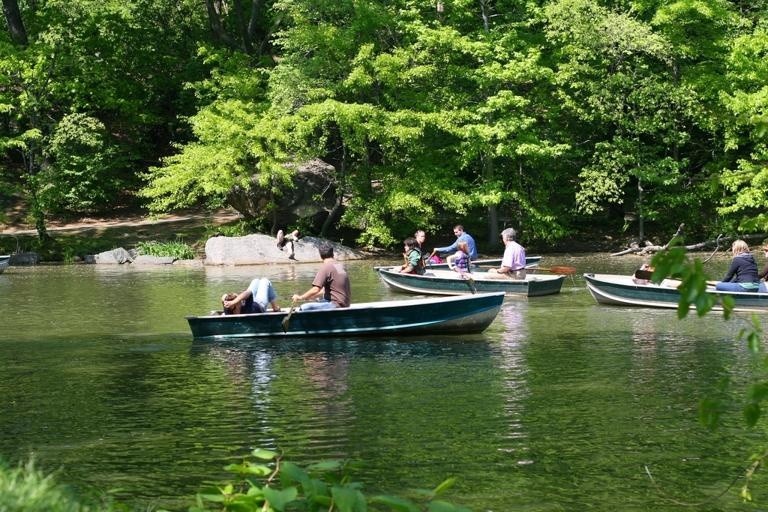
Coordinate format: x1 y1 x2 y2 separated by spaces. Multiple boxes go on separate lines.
281 301 295 333
634 269 715 286
510 266 576 273
455 267 476 295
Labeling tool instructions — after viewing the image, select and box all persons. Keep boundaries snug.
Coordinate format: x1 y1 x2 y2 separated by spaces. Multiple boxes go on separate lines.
292 244 350 311
400 237 426 276
414 230 436 263
758 243 768 293
447 240 474 274
434 224 478 261
715 239 760 292
220 277 286 315
487 228 527 280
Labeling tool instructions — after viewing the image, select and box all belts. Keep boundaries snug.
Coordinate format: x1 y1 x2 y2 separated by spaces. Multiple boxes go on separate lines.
510 268 524 271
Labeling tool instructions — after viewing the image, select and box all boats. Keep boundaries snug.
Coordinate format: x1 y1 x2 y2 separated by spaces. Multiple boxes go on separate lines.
583 273 768 312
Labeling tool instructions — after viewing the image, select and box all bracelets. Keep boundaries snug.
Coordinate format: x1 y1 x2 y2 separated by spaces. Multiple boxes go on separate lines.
302 294 306 301
